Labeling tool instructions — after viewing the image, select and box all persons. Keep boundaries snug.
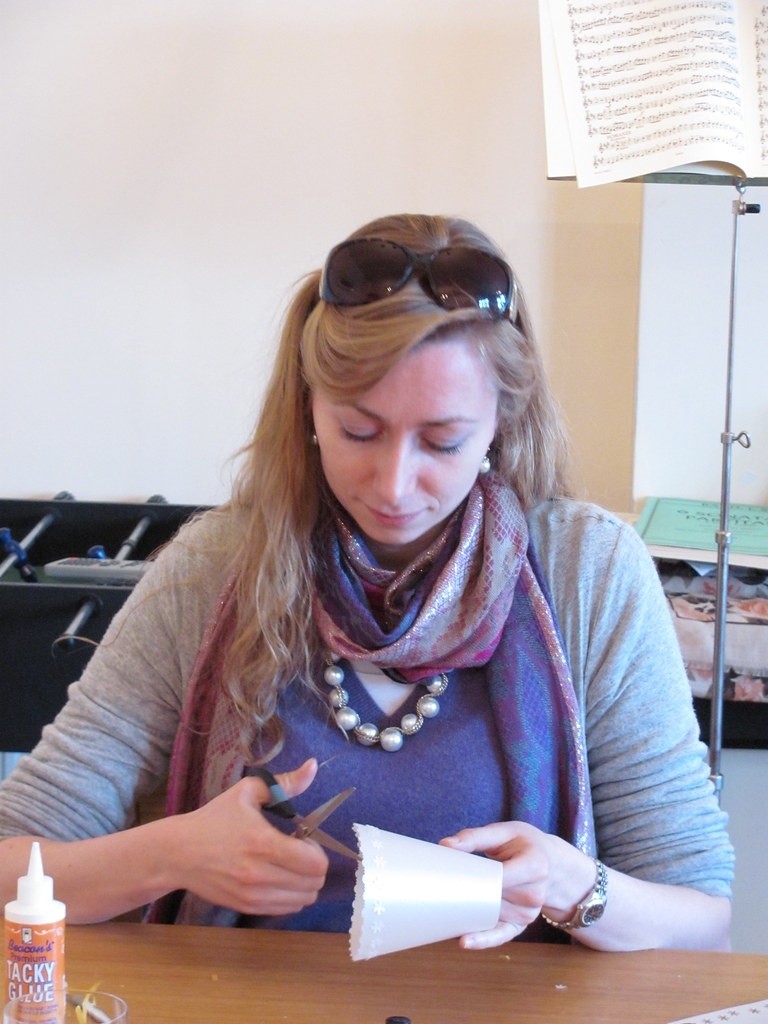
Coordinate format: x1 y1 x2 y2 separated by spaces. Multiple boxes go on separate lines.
0 214 736 954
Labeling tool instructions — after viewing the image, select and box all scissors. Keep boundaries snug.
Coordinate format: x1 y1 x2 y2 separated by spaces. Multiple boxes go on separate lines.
241 765 363 862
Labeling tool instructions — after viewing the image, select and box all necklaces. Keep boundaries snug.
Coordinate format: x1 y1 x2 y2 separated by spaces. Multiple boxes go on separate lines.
322 638 449 752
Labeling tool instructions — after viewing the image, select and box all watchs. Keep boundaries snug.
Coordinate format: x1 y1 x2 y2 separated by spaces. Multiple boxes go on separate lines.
541 857 609 932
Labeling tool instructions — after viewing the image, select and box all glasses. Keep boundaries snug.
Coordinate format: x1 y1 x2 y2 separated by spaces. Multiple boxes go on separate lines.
319 237 522 331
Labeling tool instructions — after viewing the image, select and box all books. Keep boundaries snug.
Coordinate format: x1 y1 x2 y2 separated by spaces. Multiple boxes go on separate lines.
537 0 768 188
634 496 768 570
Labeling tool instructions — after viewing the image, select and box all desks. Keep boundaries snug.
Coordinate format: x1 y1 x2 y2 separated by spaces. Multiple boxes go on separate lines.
0 921 766 1022
